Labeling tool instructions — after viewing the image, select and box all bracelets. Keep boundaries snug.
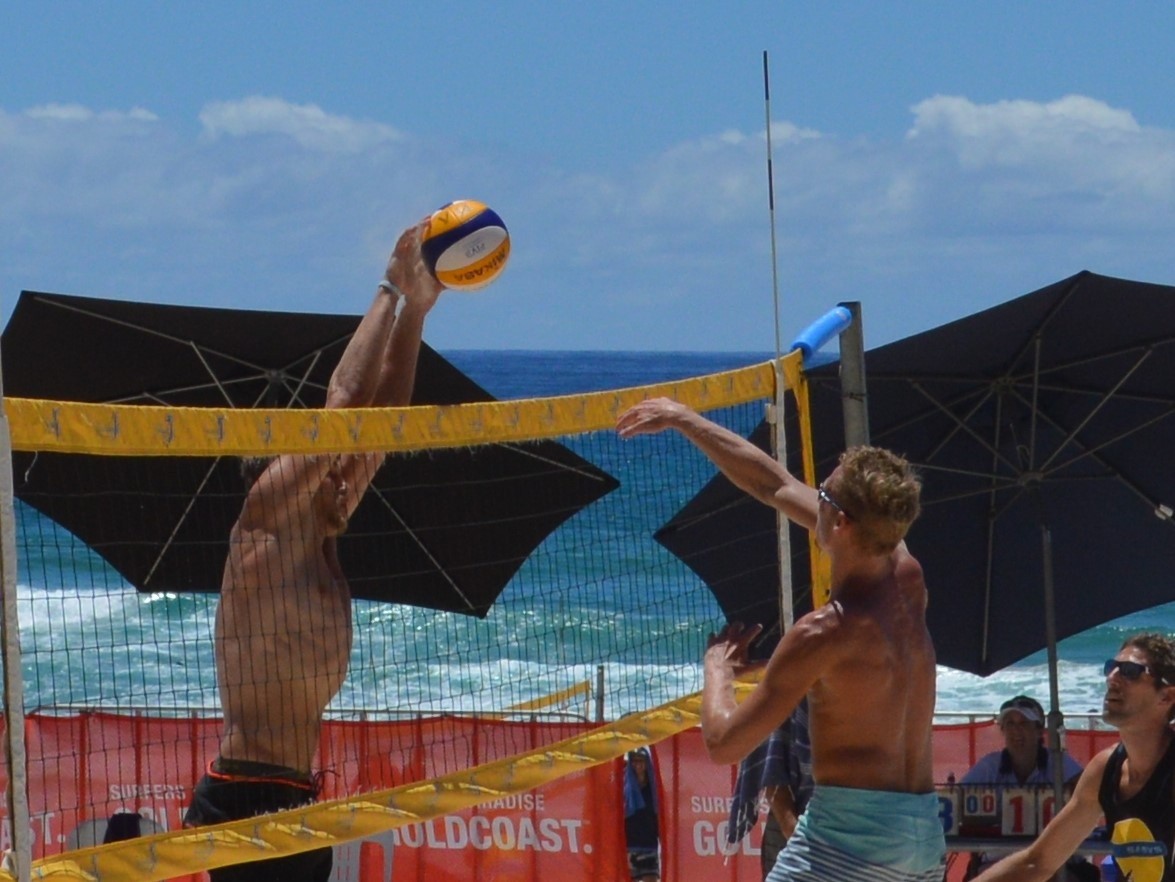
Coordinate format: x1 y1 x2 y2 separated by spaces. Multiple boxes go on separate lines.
378 281 402 299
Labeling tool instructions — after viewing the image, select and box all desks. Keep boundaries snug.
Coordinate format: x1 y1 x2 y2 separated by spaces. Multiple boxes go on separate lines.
943 831 1120 861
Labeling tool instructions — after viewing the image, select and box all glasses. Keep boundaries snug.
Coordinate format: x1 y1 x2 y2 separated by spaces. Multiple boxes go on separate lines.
817 482 854 520
1000 700 1037 709
1103 658 1172 686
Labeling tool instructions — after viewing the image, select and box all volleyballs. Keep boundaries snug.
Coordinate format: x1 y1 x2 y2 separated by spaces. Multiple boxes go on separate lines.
417 199 510 289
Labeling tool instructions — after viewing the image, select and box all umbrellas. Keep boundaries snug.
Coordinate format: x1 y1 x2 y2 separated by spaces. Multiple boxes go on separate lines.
0 290 622 620
650 270 1174 881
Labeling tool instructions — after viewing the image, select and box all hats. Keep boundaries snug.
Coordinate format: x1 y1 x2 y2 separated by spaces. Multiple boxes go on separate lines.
1000 696 1043 721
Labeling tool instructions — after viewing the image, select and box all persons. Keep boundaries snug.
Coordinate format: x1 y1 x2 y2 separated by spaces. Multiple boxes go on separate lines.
968 632 1175 882
181 215 446 882
622 746 662 882
614 395 951 882
722 693 814 881
958 695 1099 882
